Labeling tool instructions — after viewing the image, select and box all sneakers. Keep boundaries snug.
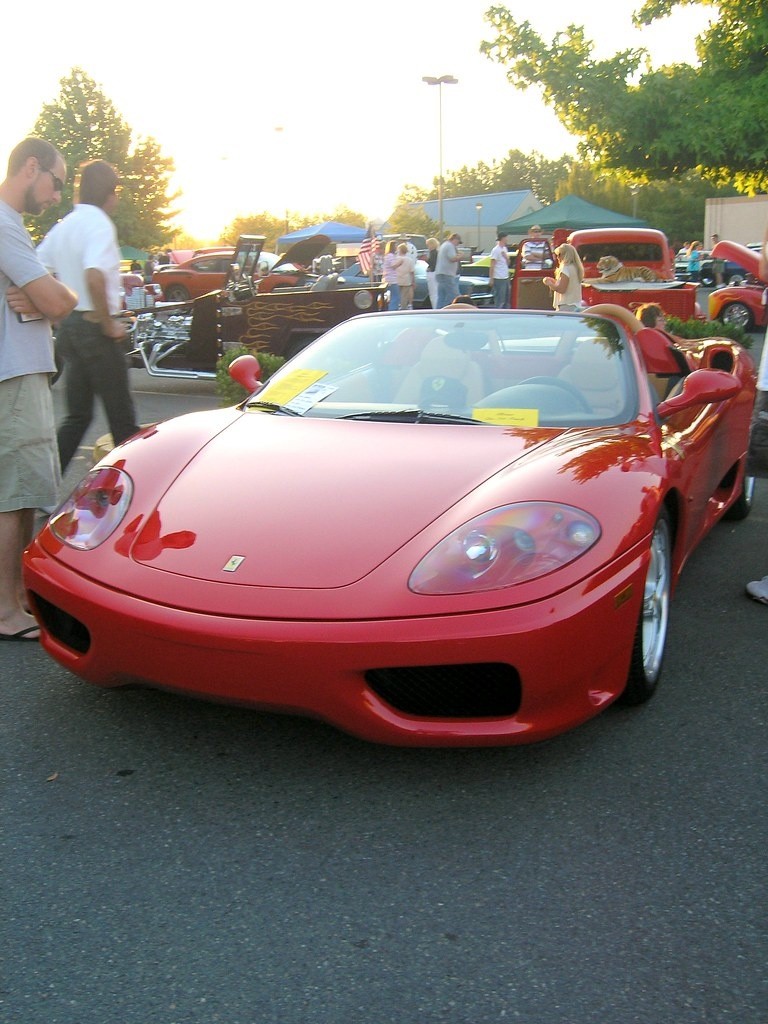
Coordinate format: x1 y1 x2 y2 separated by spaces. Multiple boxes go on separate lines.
748 581 768 603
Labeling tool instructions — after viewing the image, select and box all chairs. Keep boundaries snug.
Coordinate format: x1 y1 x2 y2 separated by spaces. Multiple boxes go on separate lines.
558 305 672 417
382 300 505 409
310 254 339 292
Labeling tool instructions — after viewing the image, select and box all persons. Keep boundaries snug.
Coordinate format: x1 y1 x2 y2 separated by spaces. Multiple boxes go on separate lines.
636 303 664 330
523 225 548 269
544 243 584 311
130 249 172 277
667 233 726 286
488 231 512 308
745 227 768 608
423 233 471 309
37 159 145 479
0 138 79 638
374 234 417 310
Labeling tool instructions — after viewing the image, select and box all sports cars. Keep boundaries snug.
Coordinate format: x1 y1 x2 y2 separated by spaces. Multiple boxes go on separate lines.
43 309 756 749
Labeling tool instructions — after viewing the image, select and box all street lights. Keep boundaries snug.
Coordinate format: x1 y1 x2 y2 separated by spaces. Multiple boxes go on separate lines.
475 202 483 252
422 73 458 244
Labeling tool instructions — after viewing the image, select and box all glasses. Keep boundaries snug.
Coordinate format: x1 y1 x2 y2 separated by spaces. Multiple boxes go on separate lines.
40 165 65 192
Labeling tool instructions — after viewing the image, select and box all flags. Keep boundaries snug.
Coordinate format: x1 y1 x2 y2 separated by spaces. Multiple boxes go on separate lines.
358 224 379 275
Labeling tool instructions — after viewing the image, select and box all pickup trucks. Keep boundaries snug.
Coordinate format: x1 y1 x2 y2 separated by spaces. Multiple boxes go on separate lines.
130 232 392 361
511 227 700 324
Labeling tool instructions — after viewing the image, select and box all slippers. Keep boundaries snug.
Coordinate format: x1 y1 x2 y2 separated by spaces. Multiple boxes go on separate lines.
0 623 41 640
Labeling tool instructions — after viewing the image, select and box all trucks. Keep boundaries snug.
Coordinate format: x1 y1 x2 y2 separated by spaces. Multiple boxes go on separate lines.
382 234 474 265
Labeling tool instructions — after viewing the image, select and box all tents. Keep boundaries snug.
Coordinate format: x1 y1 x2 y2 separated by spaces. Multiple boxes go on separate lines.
496 195 647 235
274 220 383 255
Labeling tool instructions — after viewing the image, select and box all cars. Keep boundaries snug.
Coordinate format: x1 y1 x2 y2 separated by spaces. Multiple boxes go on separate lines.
165 246 240 267
673 242 765 287
468 251 555 279
307 258 493 310
705 240 768 327
152 234 330 315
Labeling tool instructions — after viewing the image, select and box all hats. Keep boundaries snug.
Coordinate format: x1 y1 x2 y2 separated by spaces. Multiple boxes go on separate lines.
529 225 545 234
451 233 463 245
495 231 506 241
